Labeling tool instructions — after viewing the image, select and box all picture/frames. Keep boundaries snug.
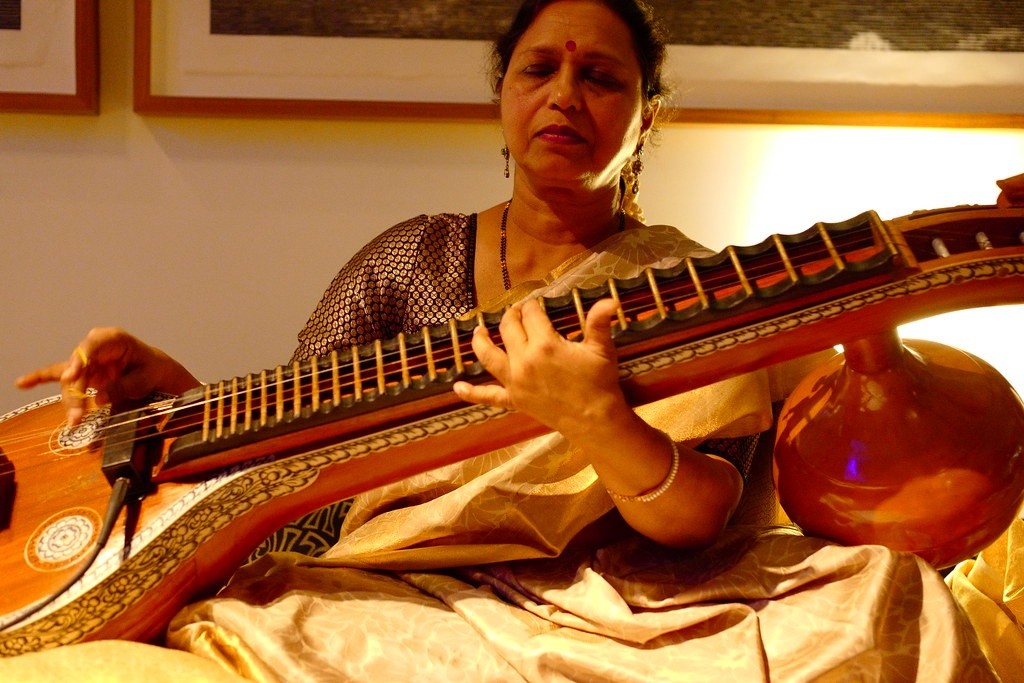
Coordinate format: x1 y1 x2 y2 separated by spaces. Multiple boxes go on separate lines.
132 0 1024 130
0 0 99 117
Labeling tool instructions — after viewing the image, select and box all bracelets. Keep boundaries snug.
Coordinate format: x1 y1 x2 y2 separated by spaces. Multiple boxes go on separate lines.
604 428 680 504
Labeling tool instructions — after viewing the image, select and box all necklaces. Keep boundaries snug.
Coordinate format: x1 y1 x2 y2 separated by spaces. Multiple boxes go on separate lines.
498 195 628 308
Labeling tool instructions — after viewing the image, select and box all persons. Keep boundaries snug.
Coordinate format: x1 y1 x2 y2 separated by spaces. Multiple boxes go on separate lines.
13 0 1000 682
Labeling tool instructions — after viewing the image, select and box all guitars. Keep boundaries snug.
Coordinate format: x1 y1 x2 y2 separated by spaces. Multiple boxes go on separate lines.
0 169 1024 655
